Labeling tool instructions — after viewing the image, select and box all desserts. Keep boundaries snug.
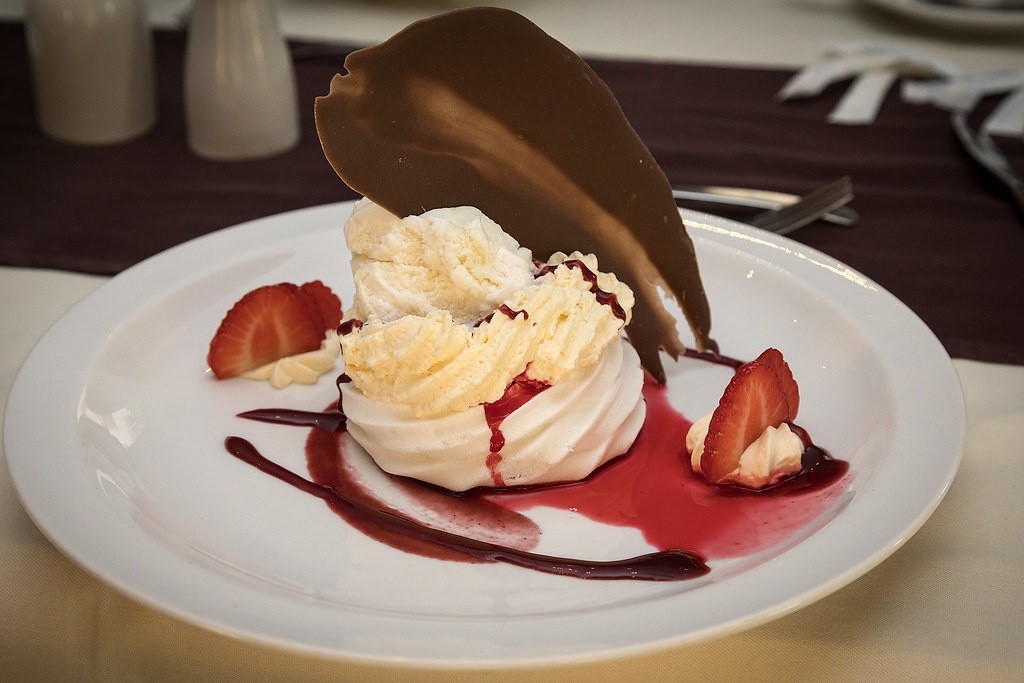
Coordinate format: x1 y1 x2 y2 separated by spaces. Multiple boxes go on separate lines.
311 5 717 497
685 347 805 492
204 277 342 389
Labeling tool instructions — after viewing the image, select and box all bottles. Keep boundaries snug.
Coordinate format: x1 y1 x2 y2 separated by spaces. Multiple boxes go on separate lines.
183 1 302 160
24 3 157 145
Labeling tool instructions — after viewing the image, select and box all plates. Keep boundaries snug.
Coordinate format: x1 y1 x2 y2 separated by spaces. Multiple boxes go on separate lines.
870 0 1024 27
5 200 968 669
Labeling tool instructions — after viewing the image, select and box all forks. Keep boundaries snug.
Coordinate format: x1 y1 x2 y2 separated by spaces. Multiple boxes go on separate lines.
748 176 853 234
